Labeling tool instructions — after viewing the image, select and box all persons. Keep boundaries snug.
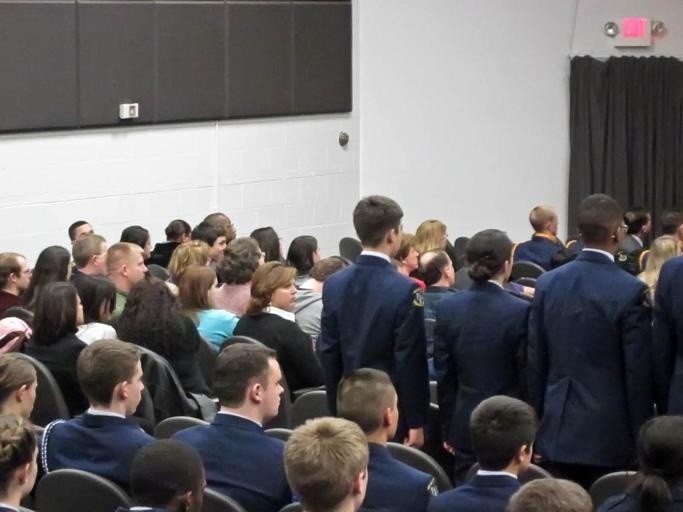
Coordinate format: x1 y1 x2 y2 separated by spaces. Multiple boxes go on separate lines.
0 194 683 512
